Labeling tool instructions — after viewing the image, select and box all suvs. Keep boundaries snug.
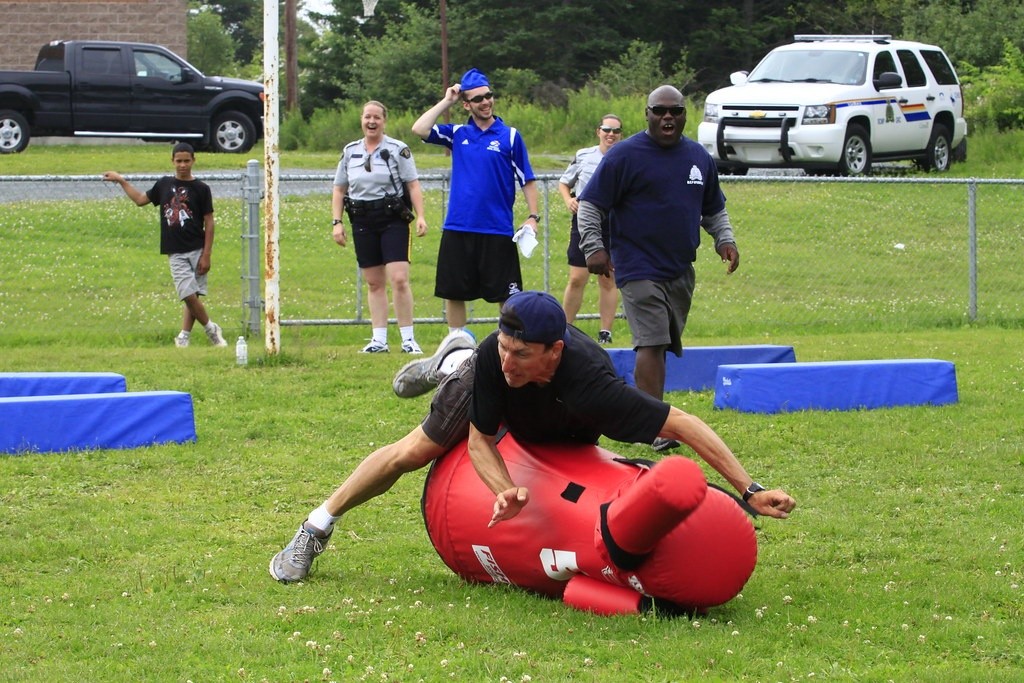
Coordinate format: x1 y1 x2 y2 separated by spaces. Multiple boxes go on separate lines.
695 34 969 179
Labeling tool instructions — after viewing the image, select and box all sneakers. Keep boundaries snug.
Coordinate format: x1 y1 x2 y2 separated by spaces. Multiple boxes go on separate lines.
207 323 227 345
176 331 191 347
268 518 335 580
360 338 390 353
653 437 681 452
391 329 475 398
596 330 612 345
402 337 424 354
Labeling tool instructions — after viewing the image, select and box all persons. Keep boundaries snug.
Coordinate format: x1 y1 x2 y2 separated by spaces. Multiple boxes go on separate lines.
412 68 538 333
576 84 740 450
104 142 227 348
559 114 623 343
333 101 428 354
269 290 797 582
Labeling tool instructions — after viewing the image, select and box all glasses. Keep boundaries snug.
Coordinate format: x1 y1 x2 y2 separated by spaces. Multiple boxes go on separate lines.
599 126 622 134
647 105 686 117
364 154 371 172
466 91 493 104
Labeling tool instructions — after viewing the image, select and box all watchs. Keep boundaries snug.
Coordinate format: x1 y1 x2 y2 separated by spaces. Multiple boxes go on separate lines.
743 482 765 502
333 219 342 225
529 215 540 222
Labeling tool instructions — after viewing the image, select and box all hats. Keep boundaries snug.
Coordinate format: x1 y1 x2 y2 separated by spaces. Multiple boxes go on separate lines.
459 67 489 92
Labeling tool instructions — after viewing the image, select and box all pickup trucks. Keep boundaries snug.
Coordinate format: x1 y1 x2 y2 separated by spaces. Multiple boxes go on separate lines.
0 38 284 154
